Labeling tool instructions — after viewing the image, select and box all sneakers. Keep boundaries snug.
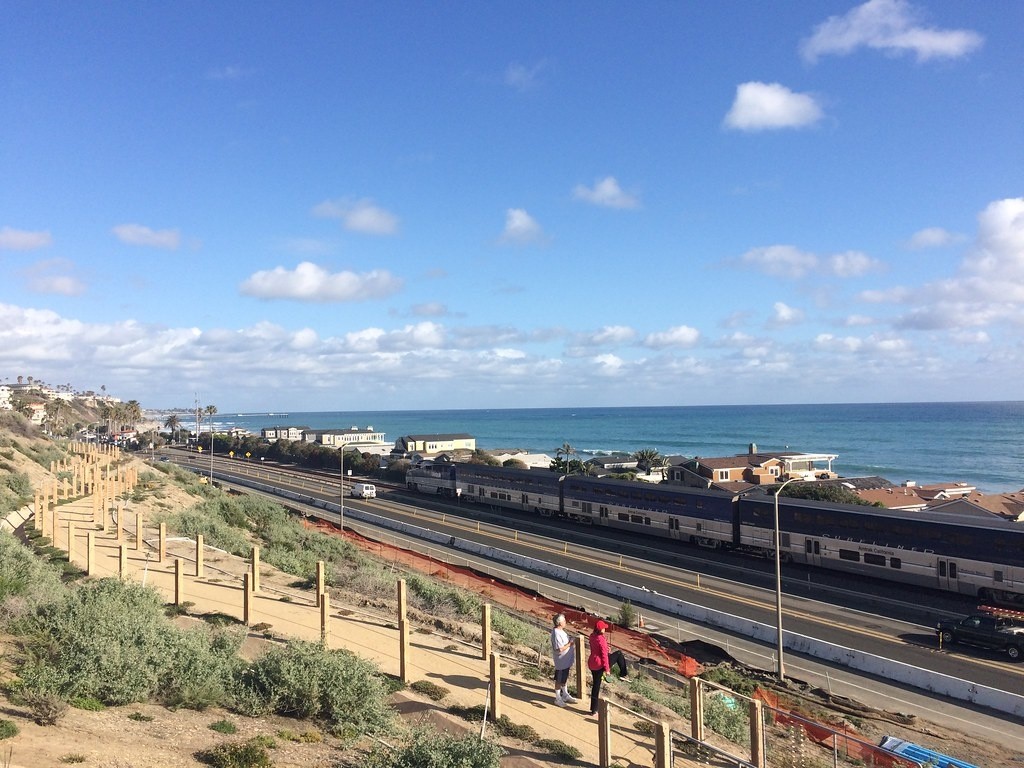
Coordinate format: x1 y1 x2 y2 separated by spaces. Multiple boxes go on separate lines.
562 694 576 703
621 674 633 684
554 697 567 707
590 708 598 715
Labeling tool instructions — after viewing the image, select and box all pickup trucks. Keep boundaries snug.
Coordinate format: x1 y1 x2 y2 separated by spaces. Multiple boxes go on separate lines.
936 612 1024 660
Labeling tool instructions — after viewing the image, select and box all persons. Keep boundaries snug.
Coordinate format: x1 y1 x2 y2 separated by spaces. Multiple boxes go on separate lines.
551 613 579 707
587 621 632 717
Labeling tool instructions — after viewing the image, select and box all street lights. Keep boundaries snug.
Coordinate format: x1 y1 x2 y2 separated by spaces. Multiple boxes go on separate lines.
772 476 818 679
340 442 363 535
210 430 230 486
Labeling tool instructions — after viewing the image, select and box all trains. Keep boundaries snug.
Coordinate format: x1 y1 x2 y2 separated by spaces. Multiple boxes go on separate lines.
405 456 1024 609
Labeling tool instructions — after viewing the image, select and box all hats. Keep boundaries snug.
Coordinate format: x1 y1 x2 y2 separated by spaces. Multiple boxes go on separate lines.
594 620 609 630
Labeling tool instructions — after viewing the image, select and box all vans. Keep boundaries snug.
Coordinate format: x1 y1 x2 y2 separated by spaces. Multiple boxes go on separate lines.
351 483 378 500
